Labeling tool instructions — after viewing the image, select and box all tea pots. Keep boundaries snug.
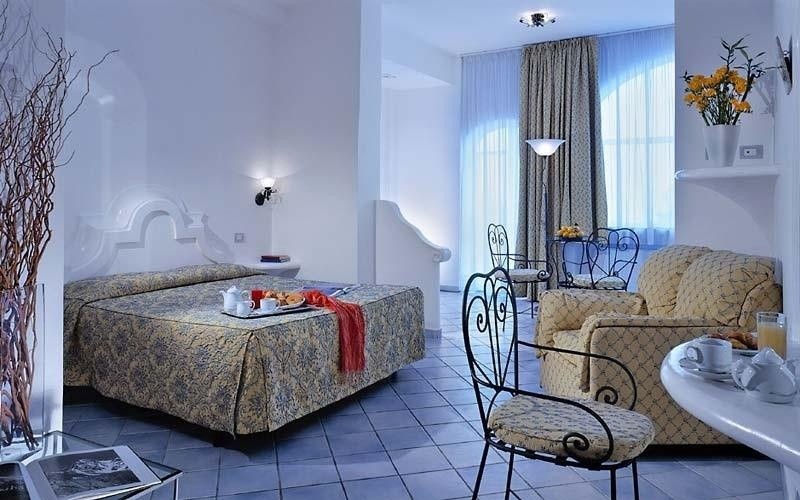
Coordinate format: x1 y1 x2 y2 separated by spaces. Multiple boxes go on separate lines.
733 347 797 404
219 285 252 311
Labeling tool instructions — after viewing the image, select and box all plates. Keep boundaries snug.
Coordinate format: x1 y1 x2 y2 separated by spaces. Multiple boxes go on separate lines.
679 357 732 380
732 331 758 356
280 297 305 309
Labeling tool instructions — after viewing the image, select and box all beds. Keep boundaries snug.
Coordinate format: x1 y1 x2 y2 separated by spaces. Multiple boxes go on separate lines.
63 186 426 452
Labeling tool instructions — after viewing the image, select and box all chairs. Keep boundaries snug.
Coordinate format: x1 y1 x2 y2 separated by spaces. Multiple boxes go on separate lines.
462 267 654 500
488 223 554 319
568 228 640 292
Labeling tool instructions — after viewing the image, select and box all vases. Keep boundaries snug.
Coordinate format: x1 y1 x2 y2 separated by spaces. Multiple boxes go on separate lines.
702 125 740 168
0 283 45 462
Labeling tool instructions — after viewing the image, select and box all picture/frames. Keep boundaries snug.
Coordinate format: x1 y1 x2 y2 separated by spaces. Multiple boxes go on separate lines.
776 36 792 96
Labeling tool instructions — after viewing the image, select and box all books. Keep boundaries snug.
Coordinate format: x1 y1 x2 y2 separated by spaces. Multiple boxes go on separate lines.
1 443 163 500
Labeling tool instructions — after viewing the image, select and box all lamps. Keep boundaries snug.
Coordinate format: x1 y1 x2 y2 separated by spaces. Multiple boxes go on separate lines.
525 139 566 311
255 177 278 206
519 11 559 28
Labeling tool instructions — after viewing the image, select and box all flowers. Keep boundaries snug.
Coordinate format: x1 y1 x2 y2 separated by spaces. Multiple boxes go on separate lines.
680 38 776 125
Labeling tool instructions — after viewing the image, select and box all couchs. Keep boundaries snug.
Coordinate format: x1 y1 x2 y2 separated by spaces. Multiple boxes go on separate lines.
535 243 782 449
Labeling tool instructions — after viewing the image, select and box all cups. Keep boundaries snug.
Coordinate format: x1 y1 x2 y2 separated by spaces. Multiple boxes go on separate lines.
685 338 732 373
237 301 255 315
260 298 280 312
757 311 786 361
